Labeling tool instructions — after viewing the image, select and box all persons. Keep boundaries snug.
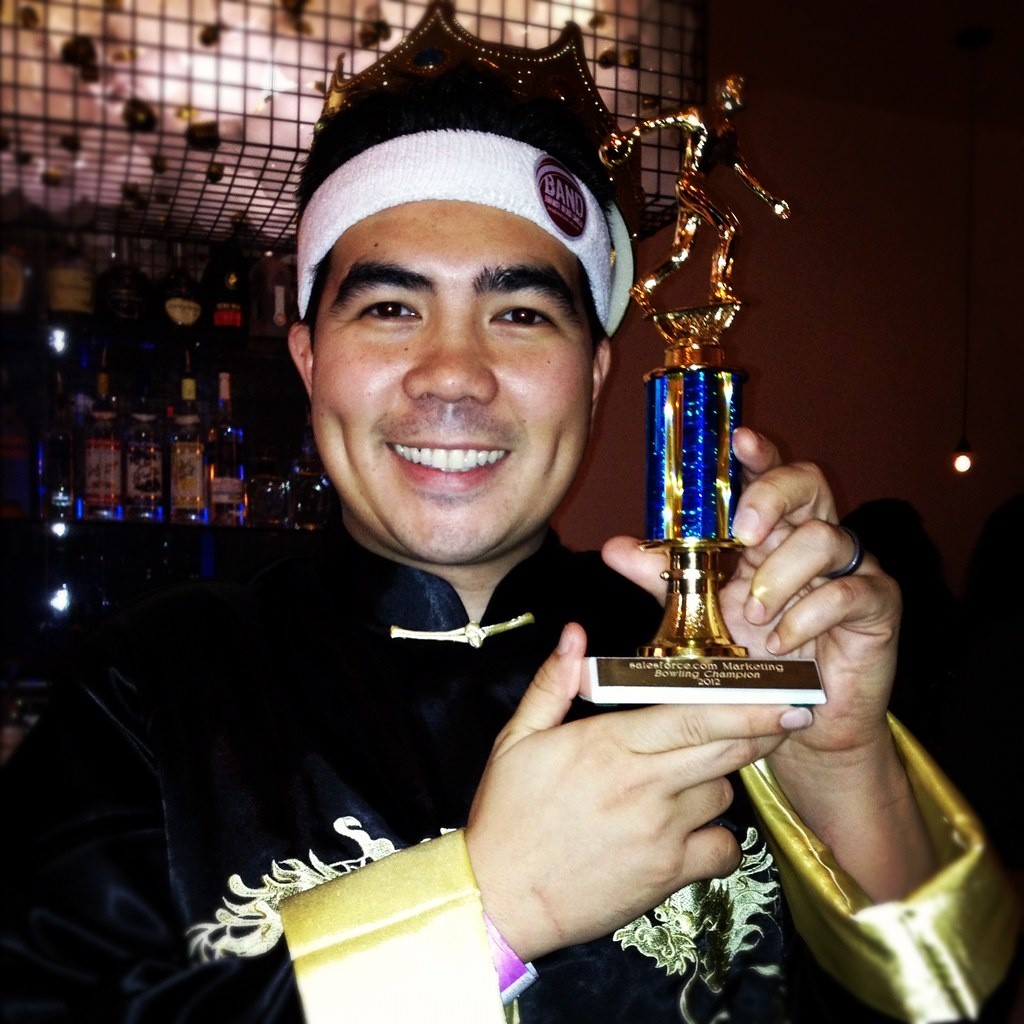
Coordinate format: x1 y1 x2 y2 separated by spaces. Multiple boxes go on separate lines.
0 3 1024 1024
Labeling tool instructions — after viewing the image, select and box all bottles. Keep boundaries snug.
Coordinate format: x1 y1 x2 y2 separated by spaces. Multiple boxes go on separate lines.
0 220 341 535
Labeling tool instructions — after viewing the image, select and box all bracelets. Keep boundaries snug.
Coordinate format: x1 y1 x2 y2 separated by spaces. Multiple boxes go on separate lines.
483 909 539 1005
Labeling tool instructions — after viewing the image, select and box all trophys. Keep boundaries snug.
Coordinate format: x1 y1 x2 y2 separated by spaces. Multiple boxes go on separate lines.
576 69 827 706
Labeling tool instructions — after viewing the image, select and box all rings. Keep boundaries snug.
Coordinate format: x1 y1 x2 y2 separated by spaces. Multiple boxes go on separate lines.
826 526 867 581
